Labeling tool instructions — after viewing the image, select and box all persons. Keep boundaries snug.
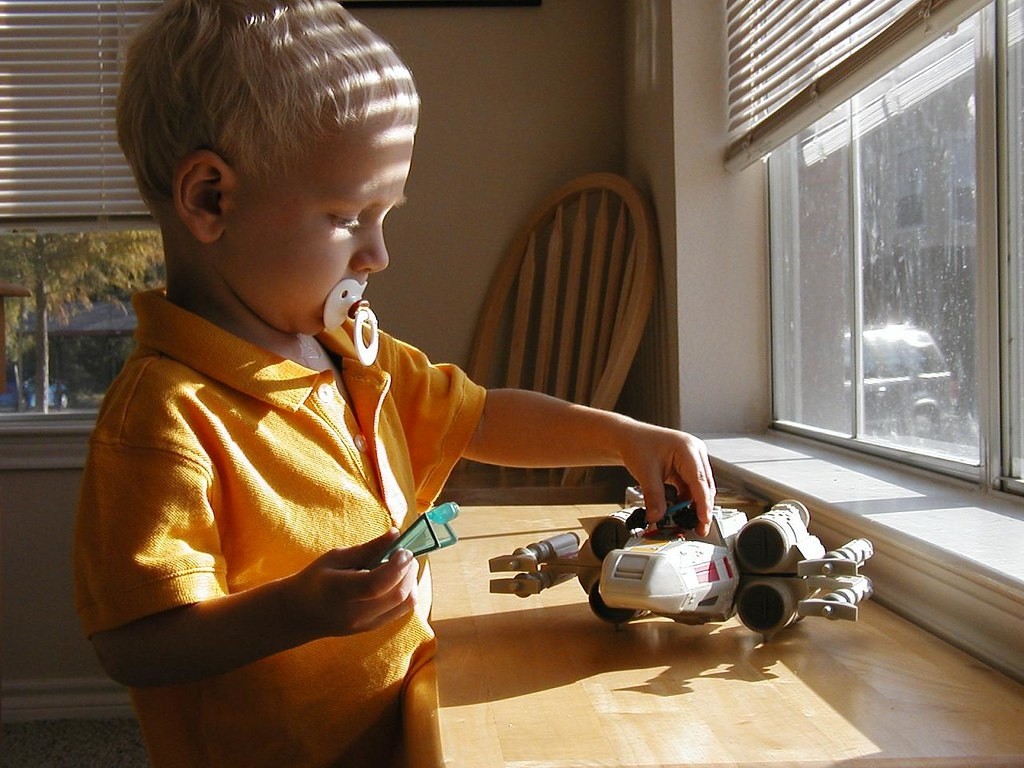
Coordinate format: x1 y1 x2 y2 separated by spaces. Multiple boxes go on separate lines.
71 1 715 768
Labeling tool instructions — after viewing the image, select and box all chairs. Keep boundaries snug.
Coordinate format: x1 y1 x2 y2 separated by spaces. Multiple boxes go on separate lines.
435 170 655 504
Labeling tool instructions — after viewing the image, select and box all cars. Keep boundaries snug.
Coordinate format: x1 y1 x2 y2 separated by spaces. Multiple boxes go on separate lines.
11 371 70 409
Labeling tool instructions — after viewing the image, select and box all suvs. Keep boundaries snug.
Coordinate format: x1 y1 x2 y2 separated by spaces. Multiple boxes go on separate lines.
839 321 964 437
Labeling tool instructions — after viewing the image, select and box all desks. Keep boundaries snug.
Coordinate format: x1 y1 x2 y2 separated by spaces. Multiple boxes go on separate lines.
401 502 1024 768
